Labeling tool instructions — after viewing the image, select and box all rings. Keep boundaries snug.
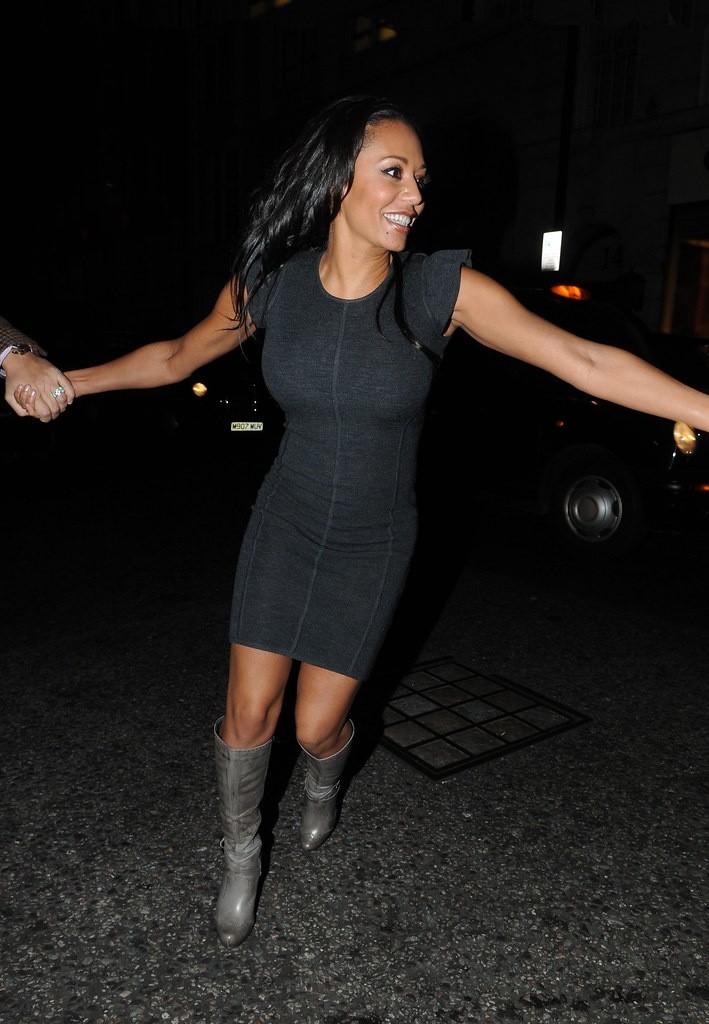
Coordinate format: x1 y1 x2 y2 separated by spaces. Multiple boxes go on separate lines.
52 387 64 398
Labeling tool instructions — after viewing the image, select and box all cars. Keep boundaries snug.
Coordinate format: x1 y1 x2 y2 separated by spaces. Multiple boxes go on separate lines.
412 279 708 562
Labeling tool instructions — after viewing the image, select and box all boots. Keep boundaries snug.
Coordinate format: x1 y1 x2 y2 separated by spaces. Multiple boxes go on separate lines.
215 716 275 949
296 718 355 852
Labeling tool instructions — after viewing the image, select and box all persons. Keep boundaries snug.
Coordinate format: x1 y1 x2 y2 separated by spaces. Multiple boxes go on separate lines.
14 92 709 950
0 315 75 424
